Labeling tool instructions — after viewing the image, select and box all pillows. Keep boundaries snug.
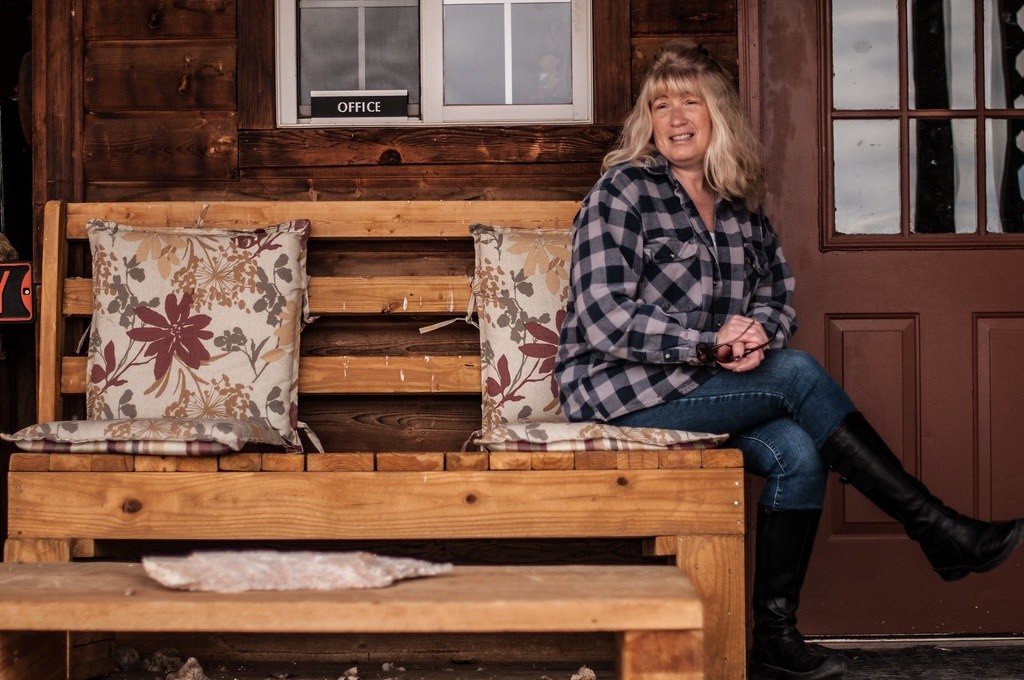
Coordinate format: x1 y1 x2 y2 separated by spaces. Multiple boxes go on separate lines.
469 224 729 452
0 216 316 456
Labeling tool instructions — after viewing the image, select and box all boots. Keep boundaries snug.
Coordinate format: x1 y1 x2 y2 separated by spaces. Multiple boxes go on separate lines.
824 408 1024 582
749 501 846 680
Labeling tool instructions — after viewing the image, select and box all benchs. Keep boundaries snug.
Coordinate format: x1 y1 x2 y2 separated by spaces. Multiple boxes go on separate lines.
0 197 747 680
0 562 706 680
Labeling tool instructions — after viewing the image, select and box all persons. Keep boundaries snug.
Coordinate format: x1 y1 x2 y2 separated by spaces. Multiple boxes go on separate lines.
552 38 1024 680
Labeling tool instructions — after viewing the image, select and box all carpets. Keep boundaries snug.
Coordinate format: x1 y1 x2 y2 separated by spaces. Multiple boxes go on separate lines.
807 643 1024 680
